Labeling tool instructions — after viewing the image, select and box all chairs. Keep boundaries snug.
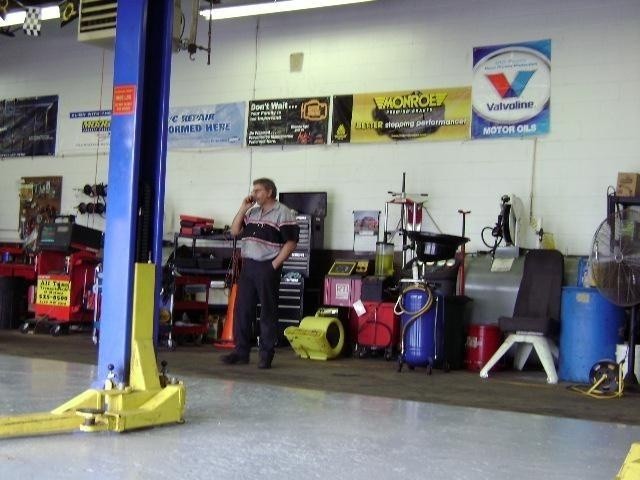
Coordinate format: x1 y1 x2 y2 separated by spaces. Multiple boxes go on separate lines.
477 247 567 386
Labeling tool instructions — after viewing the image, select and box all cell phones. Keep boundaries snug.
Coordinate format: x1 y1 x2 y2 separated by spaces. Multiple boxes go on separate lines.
250 197 256 204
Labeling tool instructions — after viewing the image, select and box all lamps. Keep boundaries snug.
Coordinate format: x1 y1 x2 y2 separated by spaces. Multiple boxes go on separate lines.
195 0 375 25
0 0 81 37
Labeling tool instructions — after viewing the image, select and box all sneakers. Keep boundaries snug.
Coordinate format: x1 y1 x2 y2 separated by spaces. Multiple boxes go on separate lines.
222 353 248 364
258 358 271 368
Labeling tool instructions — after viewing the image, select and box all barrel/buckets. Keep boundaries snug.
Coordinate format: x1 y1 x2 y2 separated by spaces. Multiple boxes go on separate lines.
463 322 504 374
556 285 627 386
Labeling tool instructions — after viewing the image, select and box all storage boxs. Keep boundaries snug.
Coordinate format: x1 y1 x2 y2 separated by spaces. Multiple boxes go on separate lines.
612 170 640 198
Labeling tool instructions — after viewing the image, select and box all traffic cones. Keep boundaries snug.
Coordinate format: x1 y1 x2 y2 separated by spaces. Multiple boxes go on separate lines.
213 281 240 350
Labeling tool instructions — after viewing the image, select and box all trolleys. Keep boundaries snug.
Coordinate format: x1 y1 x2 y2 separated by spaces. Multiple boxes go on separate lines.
18 214 103 336
352 201 401 361
90 233 212 352
396 196 475 375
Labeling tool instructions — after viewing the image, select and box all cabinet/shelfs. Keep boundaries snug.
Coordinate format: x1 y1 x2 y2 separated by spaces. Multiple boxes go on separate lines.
602 190 640 346
172 230 235 277
173 272 210 343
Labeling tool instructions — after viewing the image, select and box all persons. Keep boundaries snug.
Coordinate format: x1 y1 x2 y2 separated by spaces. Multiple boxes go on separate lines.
220 179 300 368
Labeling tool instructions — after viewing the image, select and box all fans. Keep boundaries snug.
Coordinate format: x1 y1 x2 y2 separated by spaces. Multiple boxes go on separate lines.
587 207 640 401
